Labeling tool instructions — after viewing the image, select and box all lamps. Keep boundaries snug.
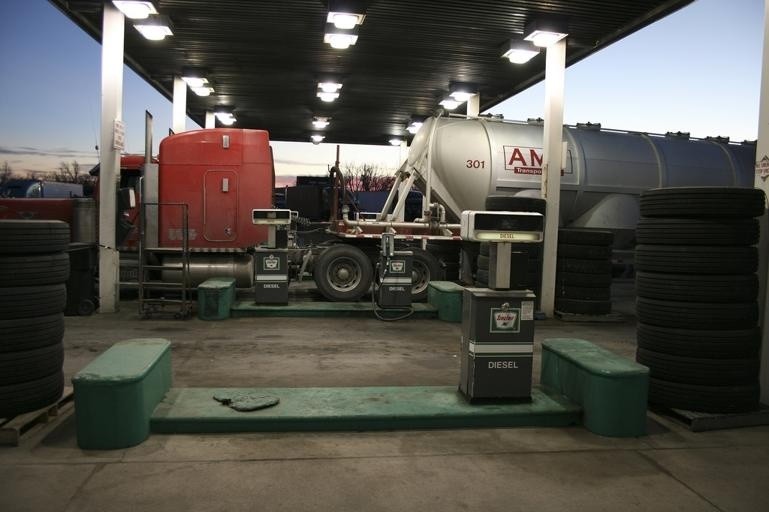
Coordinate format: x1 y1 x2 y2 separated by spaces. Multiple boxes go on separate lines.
310 0 368 144
113 1 238 126
388 19 569 147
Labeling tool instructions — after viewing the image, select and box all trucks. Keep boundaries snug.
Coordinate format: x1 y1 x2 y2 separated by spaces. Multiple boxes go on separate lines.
89 110 756 303
2 180 84 201
